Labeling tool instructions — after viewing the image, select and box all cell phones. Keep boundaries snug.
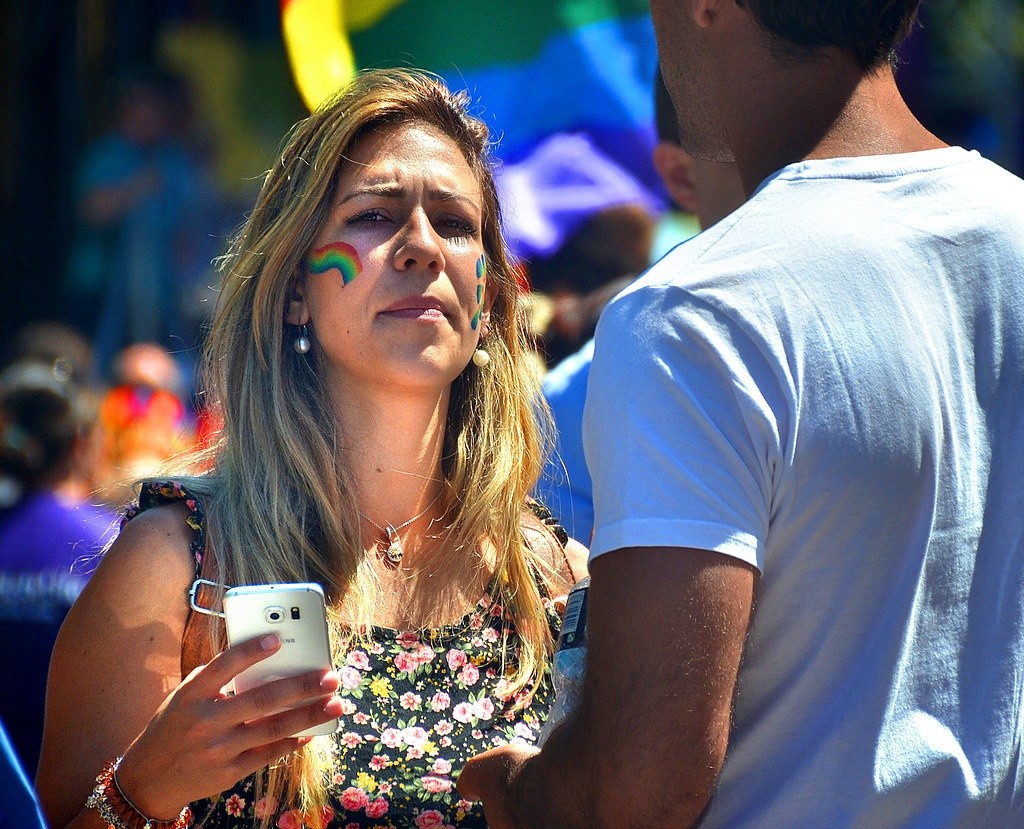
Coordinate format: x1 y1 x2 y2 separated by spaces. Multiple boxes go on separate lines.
222 582 339 738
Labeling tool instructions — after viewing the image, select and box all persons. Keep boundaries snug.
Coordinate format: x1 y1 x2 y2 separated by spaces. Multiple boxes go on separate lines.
36 66 588 829
458 1 1024 825
1 3 588 829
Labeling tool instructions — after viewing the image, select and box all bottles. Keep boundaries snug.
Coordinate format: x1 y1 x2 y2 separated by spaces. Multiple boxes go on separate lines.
539 577 593 748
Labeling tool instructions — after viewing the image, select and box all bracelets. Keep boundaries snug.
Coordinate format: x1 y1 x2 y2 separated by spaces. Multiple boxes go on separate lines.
84 756 194 829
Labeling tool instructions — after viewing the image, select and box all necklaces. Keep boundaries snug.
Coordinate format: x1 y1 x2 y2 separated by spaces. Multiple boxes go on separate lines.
356 481 445 562
